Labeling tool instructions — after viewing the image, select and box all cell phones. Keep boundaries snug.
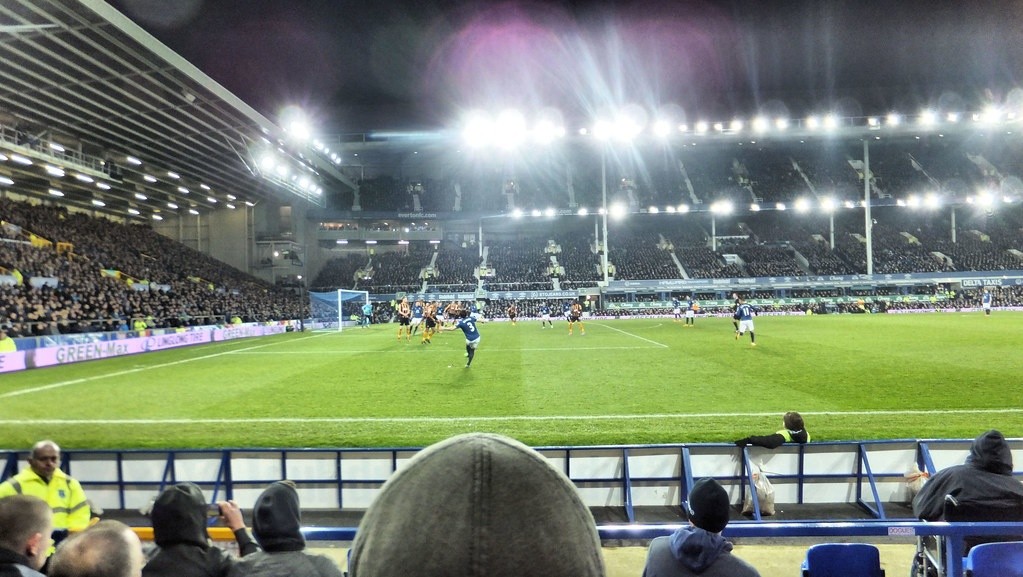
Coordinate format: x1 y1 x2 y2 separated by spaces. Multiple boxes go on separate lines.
206 505 223 516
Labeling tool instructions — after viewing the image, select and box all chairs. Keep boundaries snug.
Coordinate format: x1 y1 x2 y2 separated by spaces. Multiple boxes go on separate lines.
962 540 1023 577
799 542 885 577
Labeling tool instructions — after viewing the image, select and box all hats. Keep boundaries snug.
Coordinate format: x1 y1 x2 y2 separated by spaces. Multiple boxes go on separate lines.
688 477 729 533
348 433 606 577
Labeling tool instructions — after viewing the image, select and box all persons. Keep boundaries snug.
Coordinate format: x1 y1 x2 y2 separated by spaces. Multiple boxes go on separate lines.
0 138 1023 346
0 410 1023 577
441 309 480 368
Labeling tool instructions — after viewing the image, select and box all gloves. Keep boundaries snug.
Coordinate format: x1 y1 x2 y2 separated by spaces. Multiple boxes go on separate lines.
735 437 751 448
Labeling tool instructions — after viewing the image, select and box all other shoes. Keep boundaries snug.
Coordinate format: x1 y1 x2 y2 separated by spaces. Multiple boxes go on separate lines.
465 363 470 367
736 331 739 340
751 342 755 346
683 324 688 326
464 352 468 356
690 325 694 327
